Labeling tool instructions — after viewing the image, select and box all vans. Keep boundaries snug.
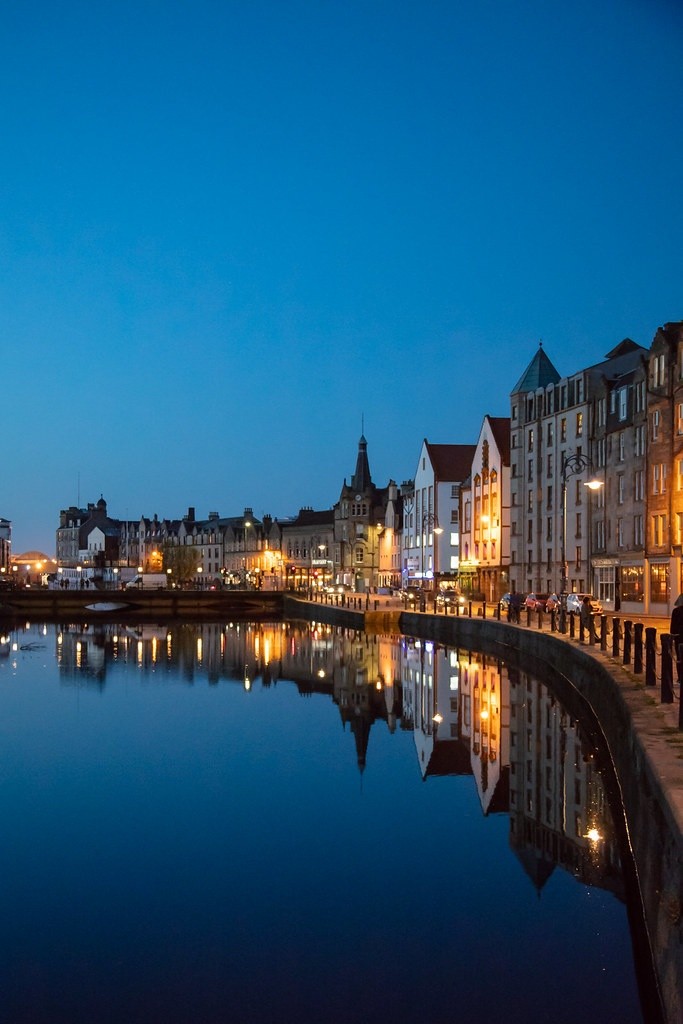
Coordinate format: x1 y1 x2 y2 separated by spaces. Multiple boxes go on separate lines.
566 594 603 616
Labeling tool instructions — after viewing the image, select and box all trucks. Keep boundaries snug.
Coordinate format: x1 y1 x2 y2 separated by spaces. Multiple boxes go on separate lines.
126 574 167 591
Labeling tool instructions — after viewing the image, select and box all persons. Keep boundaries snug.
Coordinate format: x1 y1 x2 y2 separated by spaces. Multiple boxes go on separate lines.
80 577 90 589
669 594 683 683
580 596 602 642
60 577 69 589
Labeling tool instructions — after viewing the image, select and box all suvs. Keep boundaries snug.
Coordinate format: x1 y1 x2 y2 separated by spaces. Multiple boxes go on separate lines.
400 586 425 603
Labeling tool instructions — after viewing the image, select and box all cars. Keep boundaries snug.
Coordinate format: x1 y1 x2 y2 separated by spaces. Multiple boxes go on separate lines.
525 593 550 611
500 592 525 610
329 584 352 593
436 589 467 607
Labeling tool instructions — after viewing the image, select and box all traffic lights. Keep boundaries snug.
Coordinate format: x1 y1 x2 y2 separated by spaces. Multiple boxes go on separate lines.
291 566 295 573
313 569 318 578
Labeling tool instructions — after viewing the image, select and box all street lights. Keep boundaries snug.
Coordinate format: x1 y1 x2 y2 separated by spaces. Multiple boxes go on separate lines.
167 568 172 590
138 566 144 591
197 567 202 592
419 640 442 737
420 513 444 612
242 622 251 691
245 522 250 591
77 565 82 589
310 536 326 601
114 568 119 590
309 621 326 679
559 454 606 634
